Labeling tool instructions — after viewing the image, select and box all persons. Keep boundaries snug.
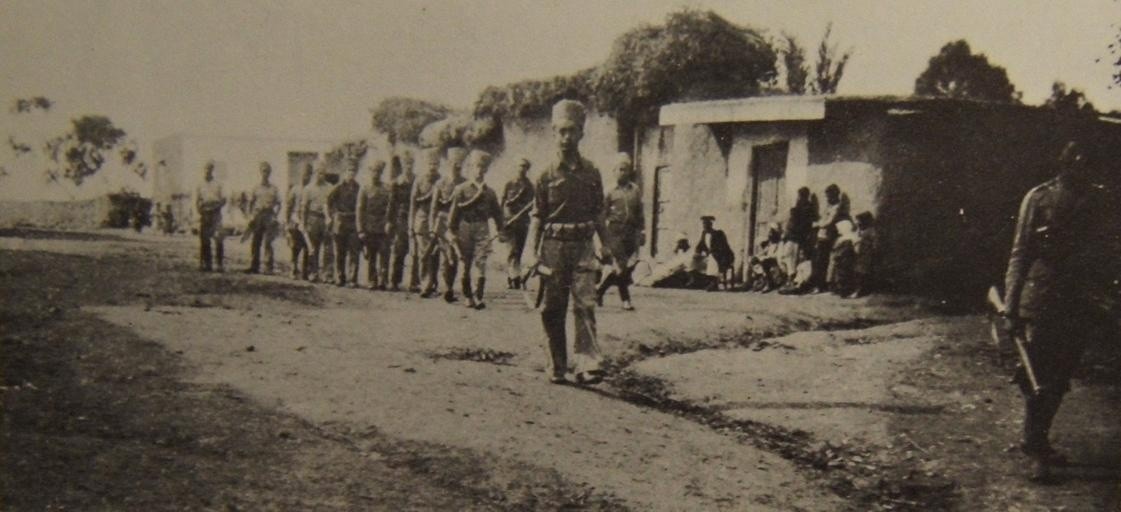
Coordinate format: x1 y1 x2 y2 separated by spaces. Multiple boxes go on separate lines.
669 182 879 299
131 198 174 237
1002 113 1121 481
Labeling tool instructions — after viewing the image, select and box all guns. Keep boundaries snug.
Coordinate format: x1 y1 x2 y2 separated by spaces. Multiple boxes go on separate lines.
437 238 455 266
529 259 555 279
445 230 463 259
988 287 1045 400
289 213 319 254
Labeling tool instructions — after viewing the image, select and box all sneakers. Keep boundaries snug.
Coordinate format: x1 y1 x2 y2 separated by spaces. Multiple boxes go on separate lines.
464 298 473 306
621 301 632 310
289 270 435 296
549 374 563 382
578 371 601 384
473 297 484 309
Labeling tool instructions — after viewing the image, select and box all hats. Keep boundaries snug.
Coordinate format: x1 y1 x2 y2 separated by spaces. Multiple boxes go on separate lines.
298 157 414 171
448 146 466 164
468 149 493 168
421 147 440 161
612 153 630 165
551 100 586 130
